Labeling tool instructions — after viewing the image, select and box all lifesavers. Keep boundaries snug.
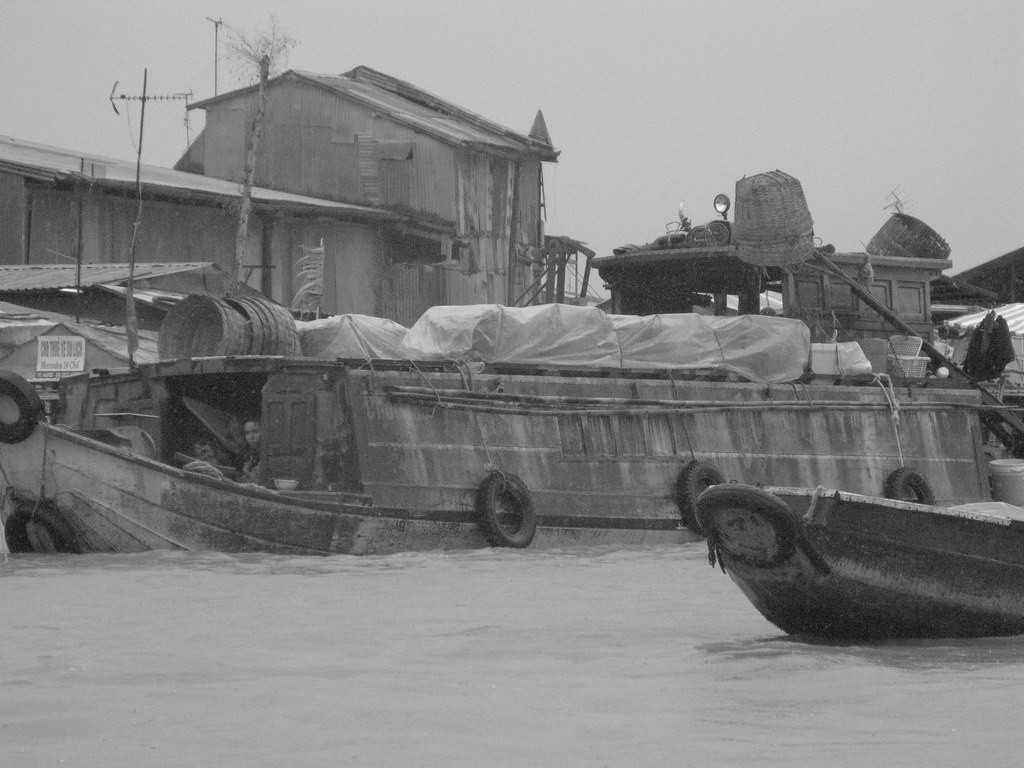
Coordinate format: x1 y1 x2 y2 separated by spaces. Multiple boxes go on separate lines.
4 502 82 555
675 461 729 538
0 369 43 444
884 466 937 507
476 472 538 549
693 482 799 568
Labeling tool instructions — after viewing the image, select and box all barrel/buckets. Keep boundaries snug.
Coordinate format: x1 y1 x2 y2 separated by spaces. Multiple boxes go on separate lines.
988 458 1024 509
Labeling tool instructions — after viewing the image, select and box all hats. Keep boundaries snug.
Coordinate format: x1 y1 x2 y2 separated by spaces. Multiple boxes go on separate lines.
241 406 261 422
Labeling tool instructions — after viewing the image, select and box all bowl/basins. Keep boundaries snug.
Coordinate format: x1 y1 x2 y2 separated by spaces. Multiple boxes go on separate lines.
274 479 299 490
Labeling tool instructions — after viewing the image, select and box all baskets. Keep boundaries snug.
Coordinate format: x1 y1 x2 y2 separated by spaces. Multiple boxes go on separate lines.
866 212 951 260
158 291 304 359
733 169 822 274
888 354 932 378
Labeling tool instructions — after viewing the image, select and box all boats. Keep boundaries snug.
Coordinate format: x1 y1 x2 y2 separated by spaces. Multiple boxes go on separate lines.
694 484 1024 643
1 246 988 554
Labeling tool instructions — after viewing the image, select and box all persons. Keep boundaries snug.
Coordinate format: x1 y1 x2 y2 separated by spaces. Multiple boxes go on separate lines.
239 417 263 483
